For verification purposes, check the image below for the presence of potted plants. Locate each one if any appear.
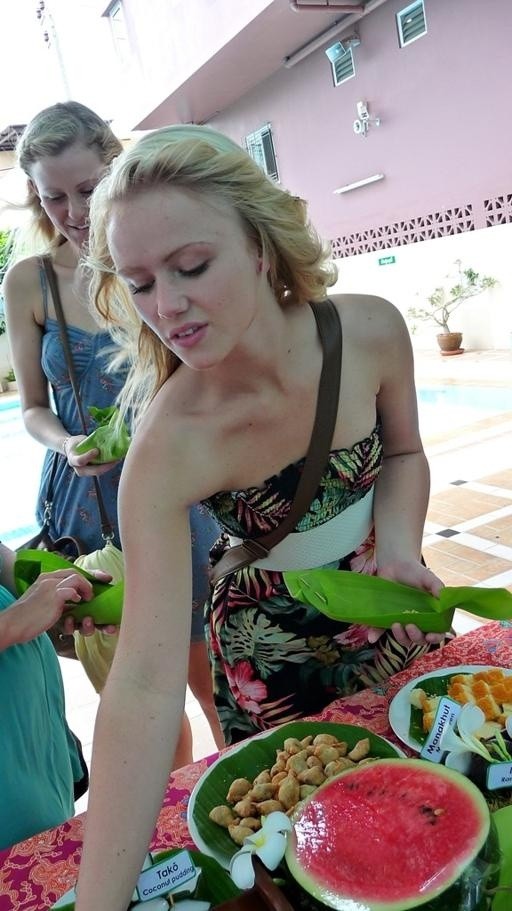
[404,260,495,350]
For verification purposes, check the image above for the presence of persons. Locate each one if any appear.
[3,100,238,774]
[73,124,446,911]
[0,537,118,857]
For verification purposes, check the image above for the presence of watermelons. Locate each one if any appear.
[285,757,501,911]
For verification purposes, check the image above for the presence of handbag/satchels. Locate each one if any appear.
[14,525,78,660]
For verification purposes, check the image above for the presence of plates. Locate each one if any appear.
[389,665,512,755]
[187,722,407,878]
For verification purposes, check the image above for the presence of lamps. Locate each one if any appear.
[325,33,360,63]
